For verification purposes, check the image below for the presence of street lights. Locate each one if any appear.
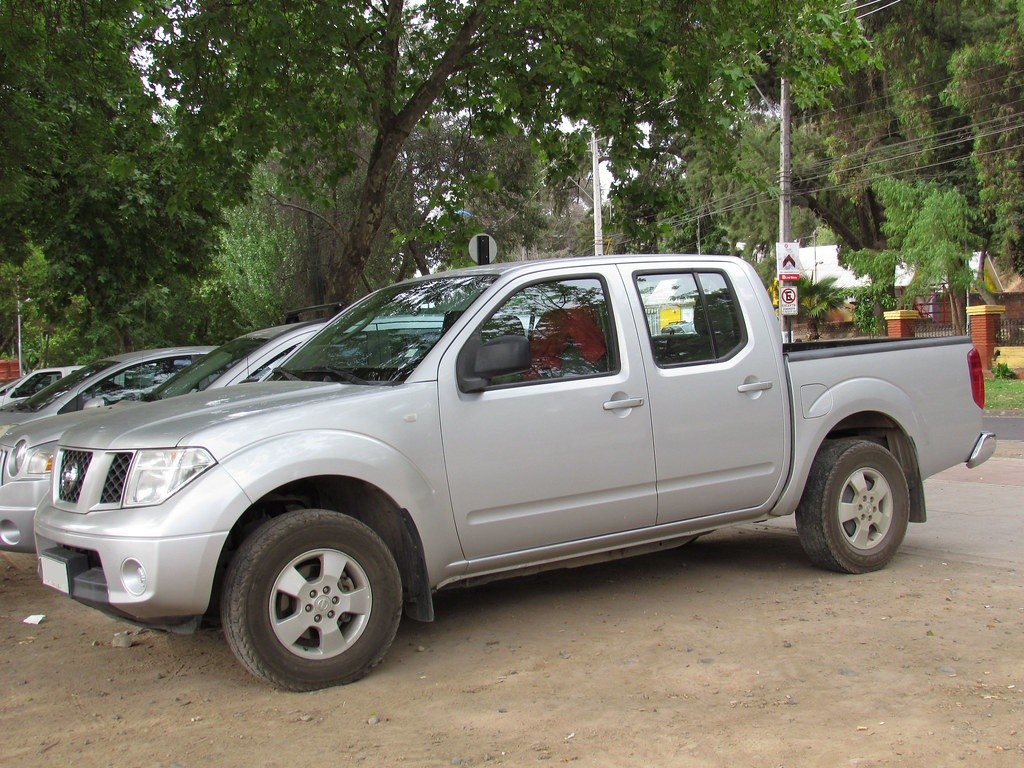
[17,285,34,376]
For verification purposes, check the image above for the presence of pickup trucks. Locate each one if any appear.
[33,253,999,693]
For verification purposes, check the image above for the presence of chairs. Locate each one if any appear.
[530,309,605,378]
[475,335,528,384]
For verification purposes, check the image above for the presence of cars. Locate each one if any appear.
[661,320,693,335]
[0,310,544,552]
[0,345,219,440]
[0,366,137,408]
